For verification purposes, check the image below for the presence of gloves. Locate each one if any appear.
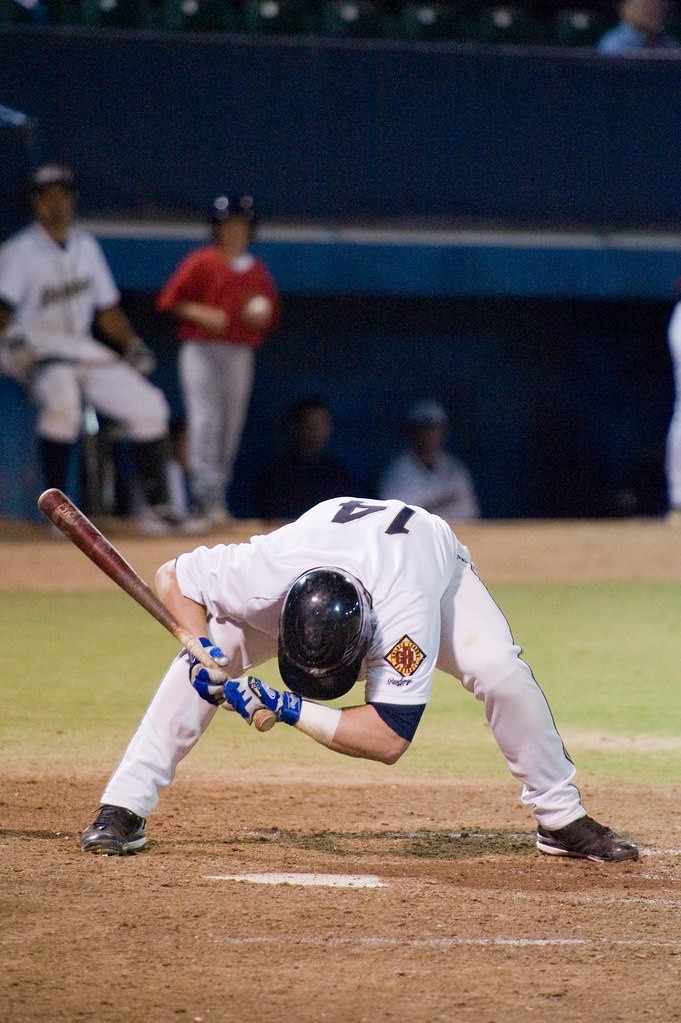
[187,637,229,706]
[6,332,35,364]
[225,676,301,727]
[124,341,155,374]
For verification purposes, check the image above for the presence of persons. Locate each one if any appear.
[378,397,481,519]
[158,198,277,533]
[0,163,213,534]
[270,395,378,518]
[76,495,640,863]
[598,0,679,52]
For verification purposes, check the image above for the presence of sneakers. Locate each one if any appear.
[79,804,149,854]
[537,817,637,863]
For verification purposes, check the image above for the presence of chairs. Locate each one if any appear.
[0,0,605,48]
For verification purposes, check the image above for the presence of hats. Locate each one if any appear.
[410,401,446,422]
[32,165,77,191]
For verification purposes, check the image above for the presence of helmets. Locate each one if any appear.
[277,565,372,700]
[210,186,260,237]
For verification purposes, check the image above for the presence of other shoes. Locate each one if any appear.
[136,508,212,536]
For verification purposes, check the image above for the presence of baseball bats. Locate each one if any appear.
[36,487,277,732]
